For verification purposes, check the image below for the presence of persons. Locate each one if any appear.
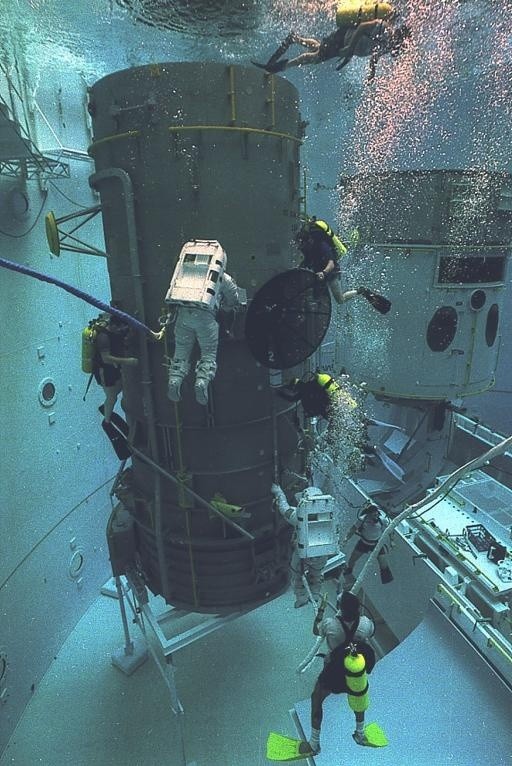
[271,481,328,608]
[93,315,138,423]
[167,272,240,405]
[306,464,314,486]
[342,506,396,575]
[280,19,412,86]
[293,220,364,304]
[308,591,376,756]
[275,373,379,454]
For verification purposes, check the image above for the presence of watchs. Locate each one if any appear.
[322,270,328,277]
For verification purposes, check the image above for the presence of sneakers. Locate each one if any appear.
[167,379,180,402]
[356,732,364,741]
[383,570,391,577]
[362,287,366,295]
[102,419,112,427]
[308,596,318,604]
[295,599,308,608]
[193,382,208,404]
[371,447,375,454]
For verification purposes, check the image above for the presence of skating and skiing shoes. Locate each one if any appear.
[298,742,317,753]
